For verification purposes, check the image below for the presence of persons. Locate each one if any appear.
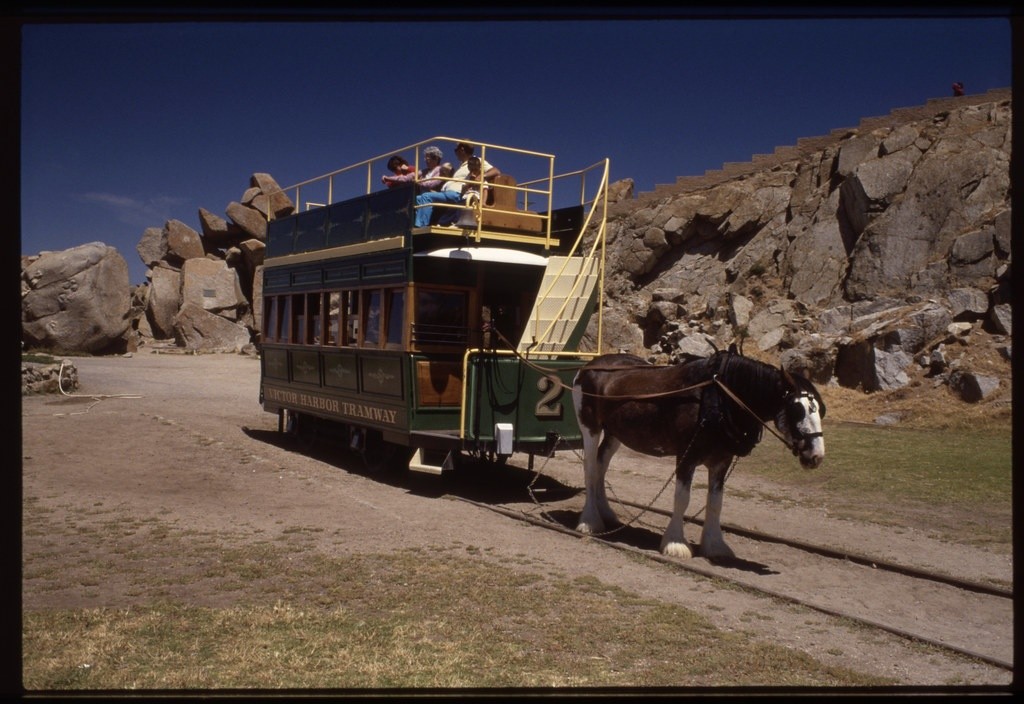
[415,136,500,227]
[381,146,443,195]
[449,157,489,230]
[440,161,454,183]
[385,155,416,188]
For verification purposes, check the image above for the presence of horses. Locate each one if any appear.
[572,350,827,560]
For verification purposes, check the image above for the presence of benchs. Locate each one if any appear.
[454,175,542,233]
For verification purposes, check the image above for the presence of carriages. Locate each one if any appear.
[259,135,827,563]
